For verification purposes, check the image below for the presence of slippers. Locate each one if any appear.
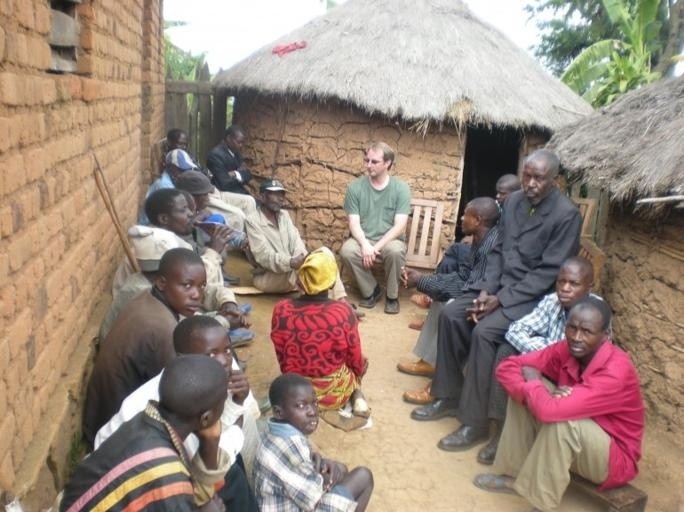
[474,474,518,494]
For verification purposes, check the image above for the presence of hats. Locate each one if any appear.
[165,148,196,170]
[259,178,287,193]
[175,172,215,196]
[296,250,337,293]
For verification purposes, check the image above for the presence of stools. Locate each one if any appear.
[567,469,648,511]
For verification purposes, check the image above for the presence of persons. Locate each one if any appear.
[408,148,582,451]
[478,256,611,464]
[474,296,645,509]
[248,374,373,510]
[269,246,372,422]
[59,121,256,511]
[399,173,521,404]
[337,139,411,316]
[241,176,362,323]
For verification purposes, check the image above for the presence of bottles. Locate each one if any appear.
[191,217,248,250]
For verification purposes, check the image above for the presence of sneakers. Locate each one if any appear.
[228,329,253,348]
[354,397,370,418]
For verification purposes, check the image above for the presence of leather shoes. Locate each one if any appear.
[360,286,382,306]
[478,434,499,463]
[438,425,489,452]
[386,296,399,314]
[399,358,457,420]
[410,319,424,329]
[411,294,432,308]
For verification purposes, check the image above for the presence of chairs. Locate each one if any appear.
[341,194,445,302]
[568,196,596,240]
[575,237,604,290]
